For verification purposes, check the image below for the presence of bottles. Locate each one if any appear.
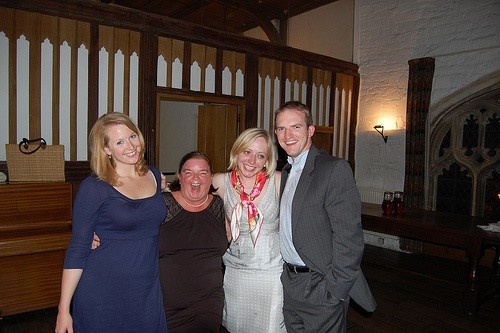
[381,191,407,218]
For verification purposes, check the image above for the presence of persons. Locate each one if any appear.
[55,112,168,333]
[91,151,232,333]
[274,101,377,333]
[161,127,287,333]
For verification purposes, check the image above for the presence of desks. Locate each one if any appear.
[360,202,500,321]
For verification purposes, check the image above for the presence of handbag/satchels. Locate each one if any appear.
[5,137,66,182]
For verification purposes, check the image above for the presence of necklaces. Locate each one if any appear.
[182,195,209,207]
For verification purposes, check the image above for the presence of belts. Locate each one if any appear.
[288,265,316,273]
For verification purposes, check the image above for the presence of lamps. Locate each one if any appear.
[374,125,388,144]
